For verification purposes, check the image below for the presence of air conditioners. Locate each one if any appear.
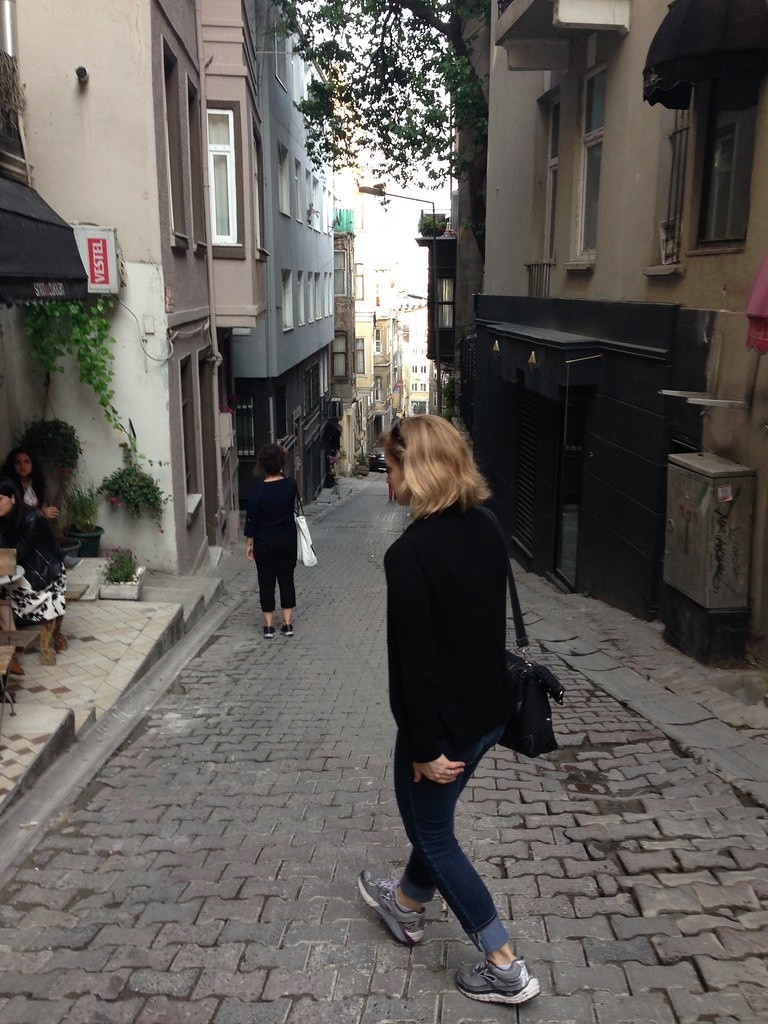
[69,221,121,296]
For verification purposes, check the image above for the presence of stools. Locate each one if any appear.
[0,644,18,716]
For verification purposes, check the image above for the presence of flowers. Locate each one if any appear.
[95,543,135,582]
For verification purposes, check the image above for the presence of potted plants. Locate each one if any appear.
[354,452,371,476]
[60,477,104,557]
[46,489,82,557]
[417,216,448,236]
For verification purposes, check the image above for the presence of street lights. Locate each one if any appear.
[357,186,443,418]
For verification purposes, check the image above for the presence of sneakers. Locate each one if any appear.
[455,956,540,1004]
[280,624,294,637]
[263,625,277,638]
[64,557,84,571]
[357,868,423,943]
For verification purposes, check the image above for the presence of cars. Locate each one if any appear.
[369,448,387,471]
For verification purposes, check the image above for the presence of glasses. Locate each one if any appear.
[392,420,407,450]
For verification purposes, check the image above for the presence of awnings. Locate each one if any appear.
[0,170,88,302]
[643,0,768,110]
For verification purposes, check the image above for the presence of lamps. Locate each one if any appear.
[491,339,509,361]
[526,350,546,375]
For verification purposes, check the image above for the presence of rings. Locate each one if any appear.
[450,770,455,776]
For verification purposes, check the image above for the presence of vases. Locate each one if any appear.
[100,564,148,599]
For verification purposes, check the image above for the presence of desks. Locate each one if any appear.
[0,582,90,666]
[0,564,26,586]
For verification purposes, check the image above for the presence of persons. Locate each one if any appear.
[243,444,297,638]
[0,449,67,675]
[356,414,541,1004]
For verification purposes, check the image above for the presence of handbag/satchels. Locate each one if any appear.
[497,647,566,758]
[296,514,317,567]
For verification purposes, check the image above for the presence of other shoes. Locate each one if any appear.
[389,500,391,502]
